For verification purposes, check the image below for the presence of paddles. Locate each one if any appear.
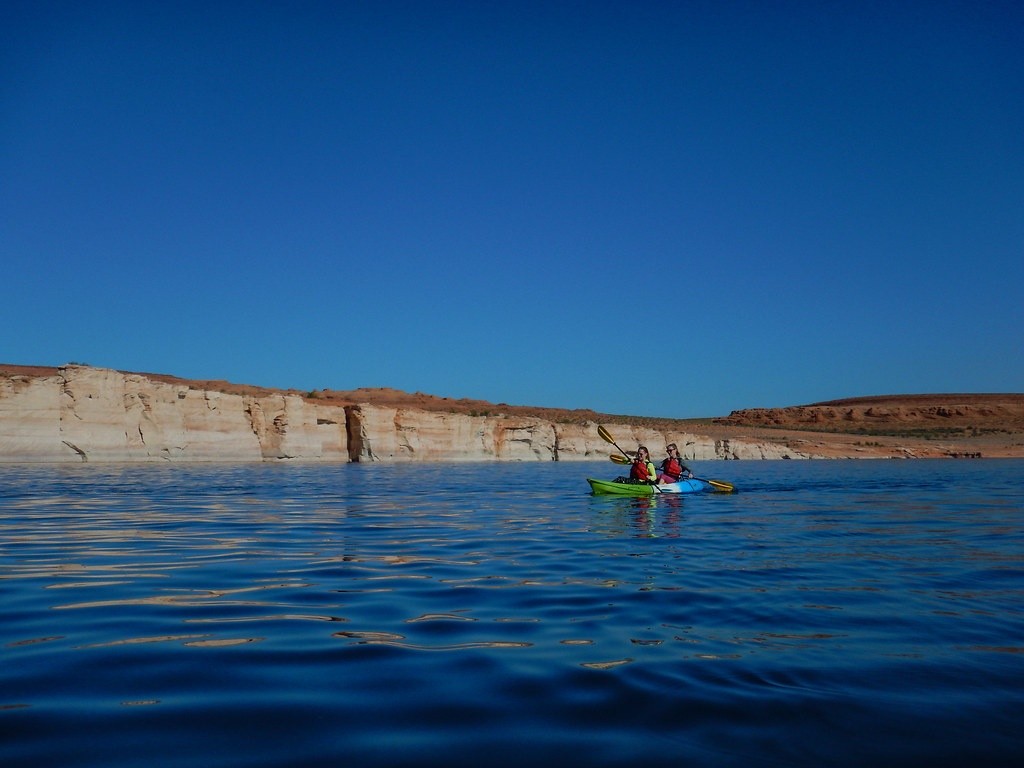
[597,425,668,497]
[610,454,733,493]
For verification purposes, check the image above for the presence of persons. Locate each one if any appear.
[659,443,693,485]
[611,447,657,485]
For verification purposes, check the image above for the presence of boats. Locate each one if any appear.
[586,477,705,499]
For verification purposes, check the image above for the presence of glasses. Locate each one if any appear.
[637,453,643,456]
[667,449,675,452]
